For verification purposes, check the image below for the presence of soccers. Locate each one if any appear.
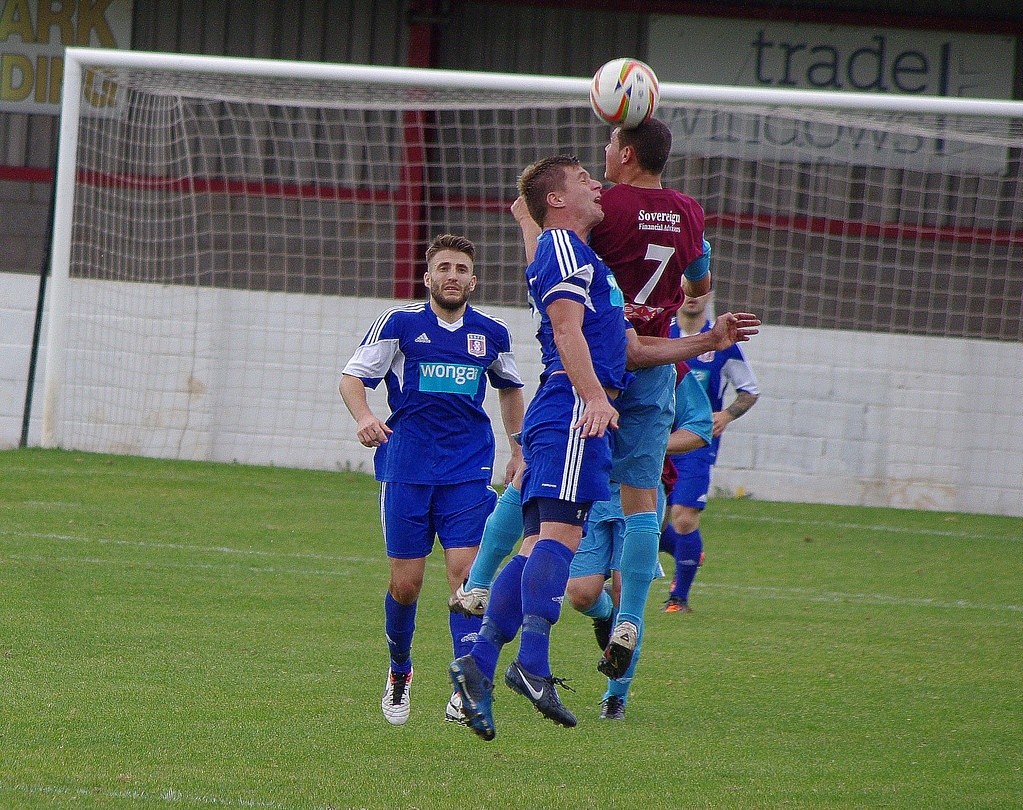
[588,56,661,130]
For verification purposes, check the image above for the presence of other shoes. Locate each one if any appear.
[661,595,688,613]
[669,551,705,597]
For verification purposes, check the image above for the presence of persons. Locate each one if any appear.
[565,359,714,721]
[450,154,761,742]
[657,289,761,611]
[449,118,711,680]
[340,234,525,726]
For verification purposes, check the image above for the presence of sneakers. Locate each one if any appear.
[591,583,614,652]
[449,654,496,741]
[598,696,625,722]
[447,578,488,620]
[597,620,637,681]
[381,666,413,726]
[445,691,471,728]
[504,657,577,728]
